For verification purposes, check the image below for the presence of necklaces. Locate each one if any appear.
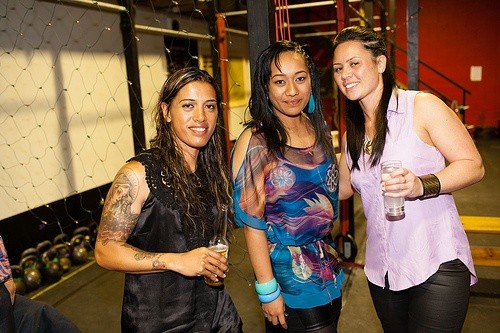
[363,95,383,153]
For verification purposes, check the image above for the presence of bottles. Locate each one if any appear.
[203,203,229,286]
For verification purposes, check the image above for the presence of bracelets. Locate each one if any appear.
[254,278,281,305]
[416,172,440,201]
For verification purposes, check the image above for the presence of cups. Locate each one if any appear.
[381,160,405,221]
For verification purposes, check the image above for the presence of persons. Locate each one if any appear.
[12,293,83,332]
[0,235,18,333]
[230,41,351,332]
[95,67,245,332]
[332,26,486,333]
